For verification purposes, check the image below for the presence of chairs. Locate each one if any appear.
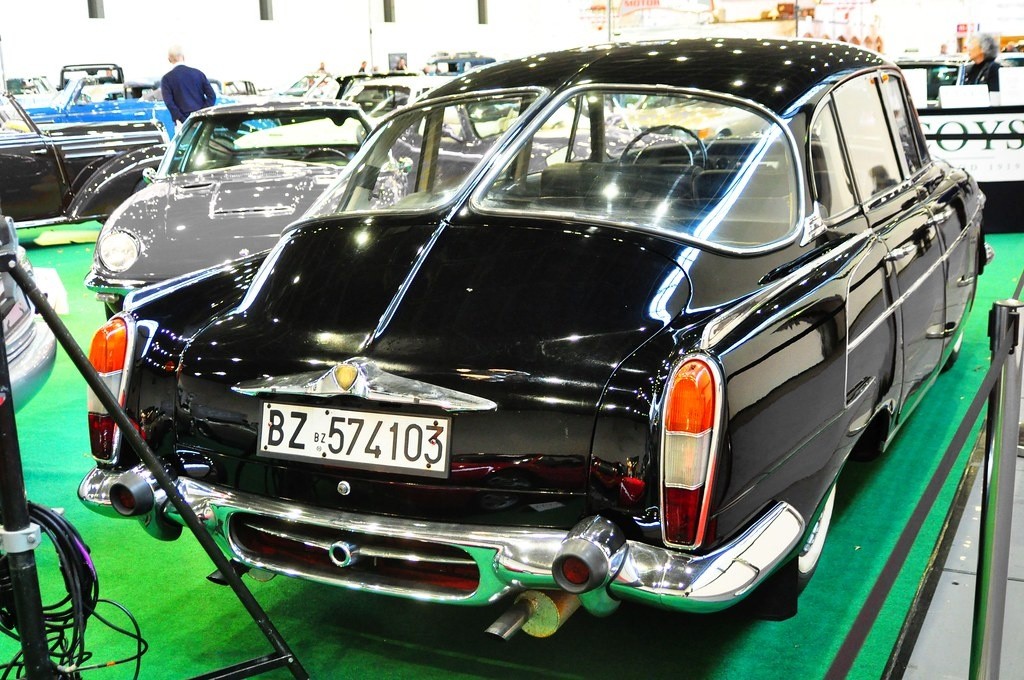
[545,163,703,205]
[690,169,850,203]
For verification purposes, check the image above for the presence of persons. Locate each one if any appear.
[964,33,1005,91]
[161,45,217,144]
[359,58,407,72]
[319,62,325,70]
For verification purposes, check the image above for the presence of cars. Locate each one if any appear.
[612,96,772,139]
[22,76,276,142]
[891,52,1024,107]
[0,91,185,230]
[231,75,645,166]
[82,99,412,322]
[75,37,994,641]
